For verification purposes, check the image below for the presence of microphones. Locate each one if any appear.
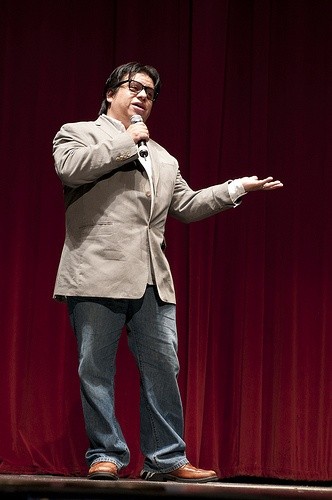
[130,115,148,158]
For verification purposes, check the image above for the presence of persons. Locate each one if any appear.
[52,62,283,483]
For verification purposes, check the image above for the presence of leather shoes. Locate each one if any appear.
[140,462,218,483]
[87,462,119,480]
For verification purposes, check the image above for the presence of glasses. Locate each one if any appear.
[114,79,159,101]
